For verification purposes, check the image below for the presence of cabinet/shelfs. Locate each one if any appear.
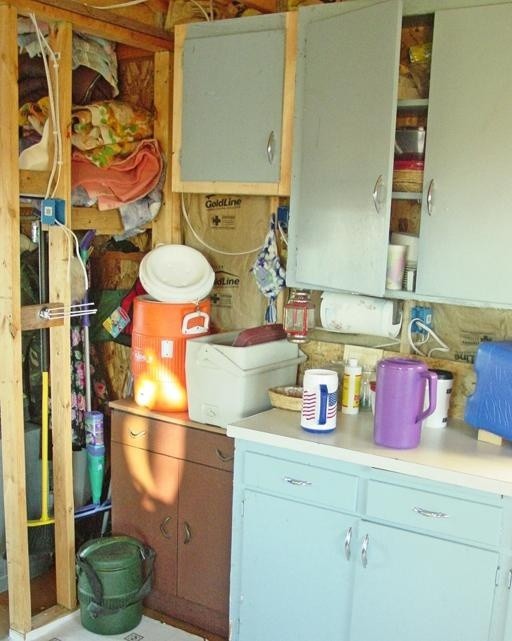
[170,11,292,199]
[286,0,512,312]
[108,398,235,641]
[229,438,511,639]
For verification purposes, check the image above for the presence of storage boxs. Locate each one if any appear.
[184,329,308,429]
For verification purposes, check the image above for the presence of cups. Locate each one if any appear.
[373,357,438,450]
[302,368,338,434]
[421,370,454,430]
[361,379,373,411]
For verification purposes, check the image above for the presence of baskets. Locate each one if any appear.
[392,169,424,193]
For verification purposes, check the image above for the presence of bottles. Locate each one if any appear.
[342,358,362,415]
[386,245,415,291]
[283,292,315,343]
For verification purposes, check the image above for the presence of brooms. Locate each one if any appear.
[4,218,57,560]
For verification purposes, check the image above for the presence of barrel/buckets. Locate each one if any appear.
[131,243,216,414]
[76,536,146,634]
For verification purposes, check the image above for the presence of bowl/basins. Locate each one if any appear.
[390,231,420,262]
